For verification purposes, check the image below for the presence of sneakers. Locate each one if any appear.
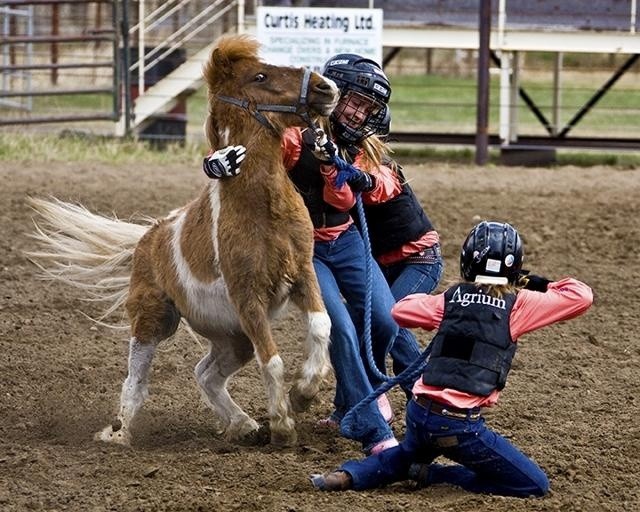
[372,438,398,456]
[376,393,392,423]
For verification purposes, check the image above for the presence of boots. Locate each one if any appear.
[312,470,351,490]
[407,462,438,483]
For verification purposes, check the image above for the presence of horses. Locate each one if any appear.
[19,34,342,450]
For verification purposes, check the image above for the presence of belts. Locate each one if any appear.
[412,395,481,422]
[403,244,441,265]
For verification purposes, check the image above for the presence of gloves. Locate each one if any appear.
[202,145,246,178]
[303,129,338,165]
[329,153,375,194]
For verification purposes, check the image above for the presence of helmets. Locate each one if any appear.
[460,222,522,287]
[366,105,390,138]
[322,53,391,143]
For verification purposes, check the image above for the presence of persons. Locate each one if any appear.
[201,53,401,454]
[309,221,595,496]
[315,103,441,429]
